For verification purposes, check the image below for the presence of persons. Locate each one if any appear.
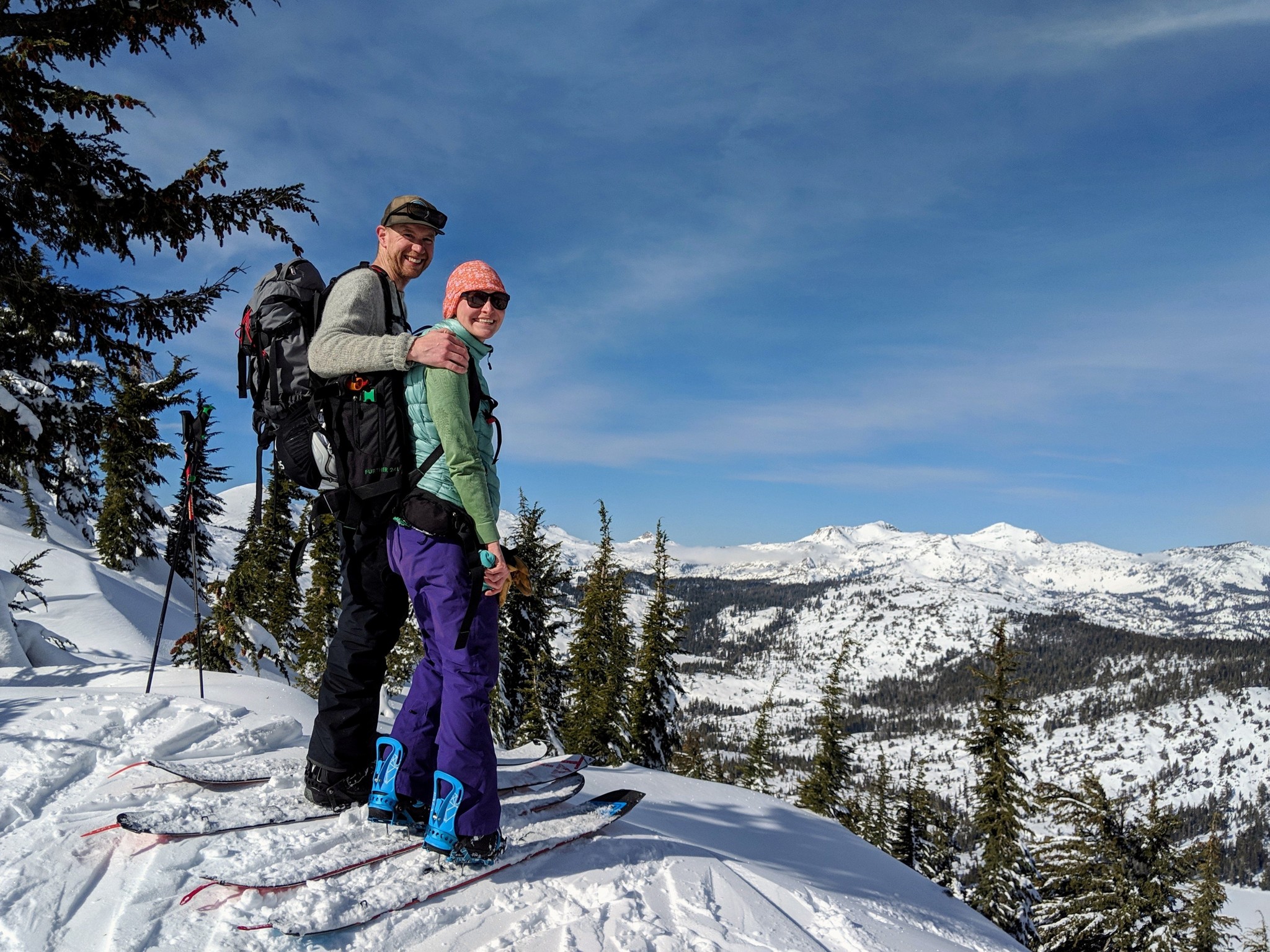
[367,258,515,868]
[304,195,471,813]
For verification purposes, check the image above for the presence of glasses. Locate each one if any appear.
[383,199,448,229]
[460,290,511,311]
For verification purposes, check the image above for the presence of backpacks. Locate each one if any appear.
[324,332,502,527]
[237,257,405,490]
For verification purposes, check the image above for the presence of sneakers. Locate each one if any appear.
[368,791,433,825]
[303,760,376,812]
[425,833,507,865]
[376,731,391,758]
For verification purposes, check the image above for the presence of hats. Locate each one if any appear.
[381,195,446,236]
[442,260,506,320]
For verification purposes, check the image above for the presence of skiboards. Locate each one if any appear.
[113,738,597,836]
[185,774,649,938]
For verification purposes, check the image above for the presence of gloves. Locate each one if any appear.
[499,555,533,607]
